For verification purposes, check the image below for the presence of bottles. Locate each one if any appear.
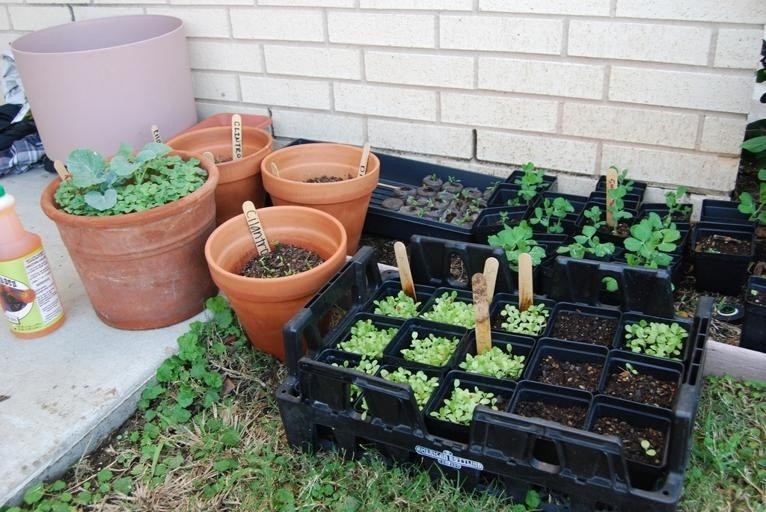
[0,179,68,339]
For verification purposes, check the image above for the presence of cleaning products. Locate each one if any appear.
[0,183,63,338]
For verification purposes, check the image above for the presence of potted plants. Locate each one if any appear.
[42,141,220,330]
